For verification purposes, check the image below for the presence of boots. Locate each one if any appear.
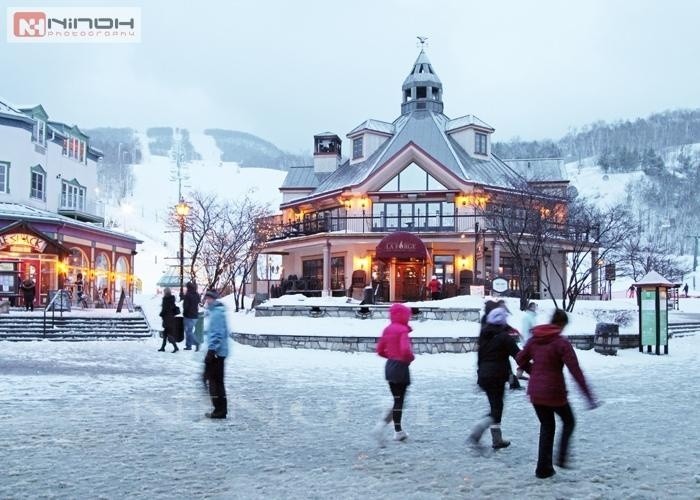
[466,424,484,446]
[158,340,167,352]
[491,426,510,448]
[171,343,178,353]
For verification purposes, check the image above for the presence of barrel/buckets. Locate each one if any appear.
[594,323,620,354]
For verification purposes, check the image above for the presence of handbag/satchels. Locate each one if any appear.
[173,305,180,315]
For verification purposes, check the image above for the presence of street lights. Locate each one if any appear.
[174,195,190,302]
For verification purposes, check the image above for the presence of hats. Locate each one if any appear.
[486,307,507,326]
[205,289,218,298]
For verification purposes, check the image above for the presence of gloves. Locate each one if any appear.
[205,349,215,364]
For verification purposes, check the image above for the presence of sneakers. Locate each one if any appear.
[206,412,225,418]
[372,425,387,447]
[394,430,408,440]
[184,346,191,350]
[511,386,525,390]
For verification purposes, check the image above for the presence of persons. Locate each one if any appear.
[460,307,532,454]
[22,275,36,311]
[159,288,180,353]
[513,310,603,479]
[369,303,414,448]
[200,289,230,419]
[428,274,442,300]
[481,298,540,394]
[683,283,689,298]
[182,281,202,353]
[627,285,636,298]
